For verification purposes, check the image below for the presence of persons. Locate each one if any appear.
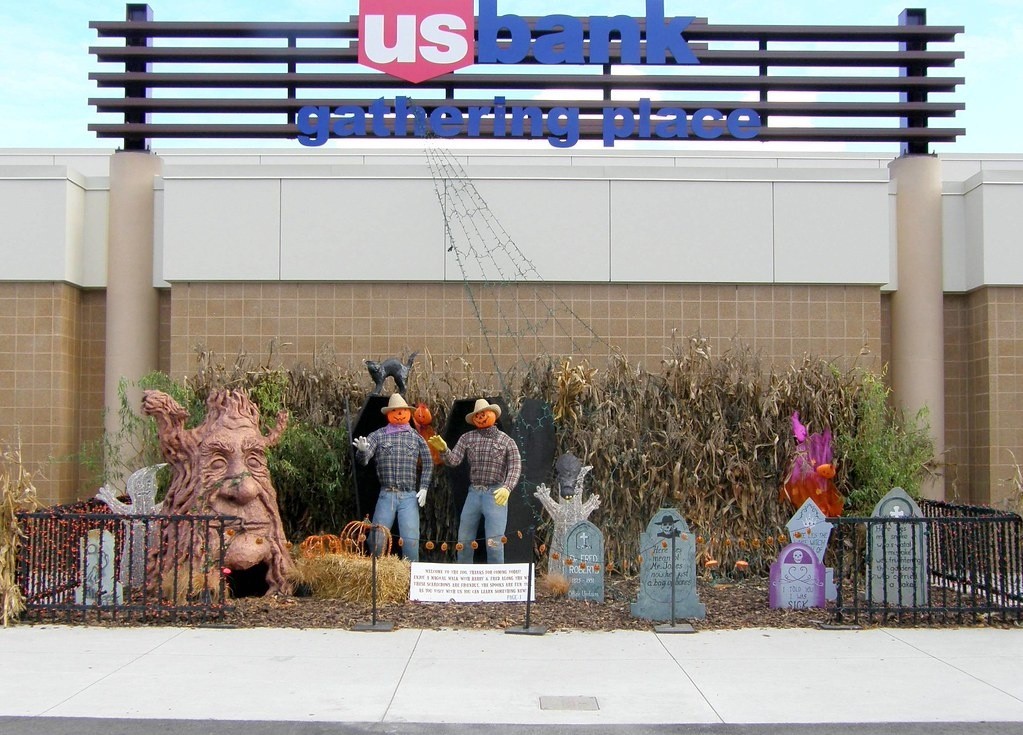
[352,392,433,562]
[426,398,521,564]
[141,383,299,597]
